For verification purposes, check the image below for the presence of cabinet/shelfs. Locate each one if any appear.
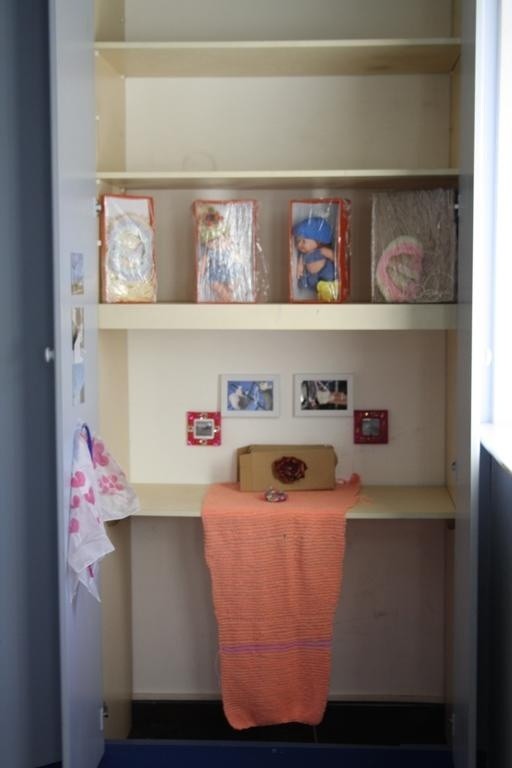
[43,0,482,768]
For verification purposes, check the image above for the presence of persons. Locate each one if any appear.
[289,213,341,305]
[197,204,250,301]
